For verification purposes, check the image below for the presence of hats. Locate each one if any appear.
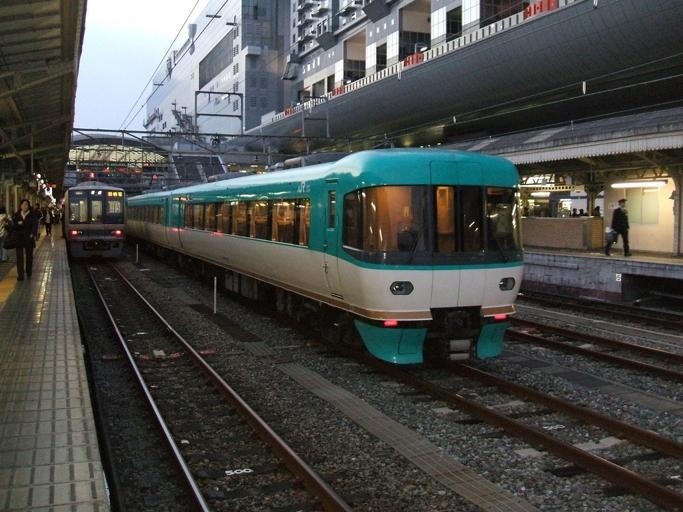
[618,199,629,202]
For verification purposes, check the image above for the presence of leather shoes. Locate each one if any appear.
[605,249,611,256]
[625,253,633,257]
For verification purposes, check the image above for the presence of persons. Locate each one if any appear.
[604,199,632,256]
[571,206,601,216]
[0,199,38,281]
[34,203,65,240]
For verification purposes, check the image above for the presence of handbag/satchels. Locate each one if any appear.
[605,231,618,242]
[2,230,36,249]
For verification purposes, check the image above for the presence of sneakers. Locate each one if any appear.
[16,271,32,282]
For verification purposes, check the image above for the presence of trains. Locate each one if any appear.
[127,147,525,366]
[62,181,127,265]
